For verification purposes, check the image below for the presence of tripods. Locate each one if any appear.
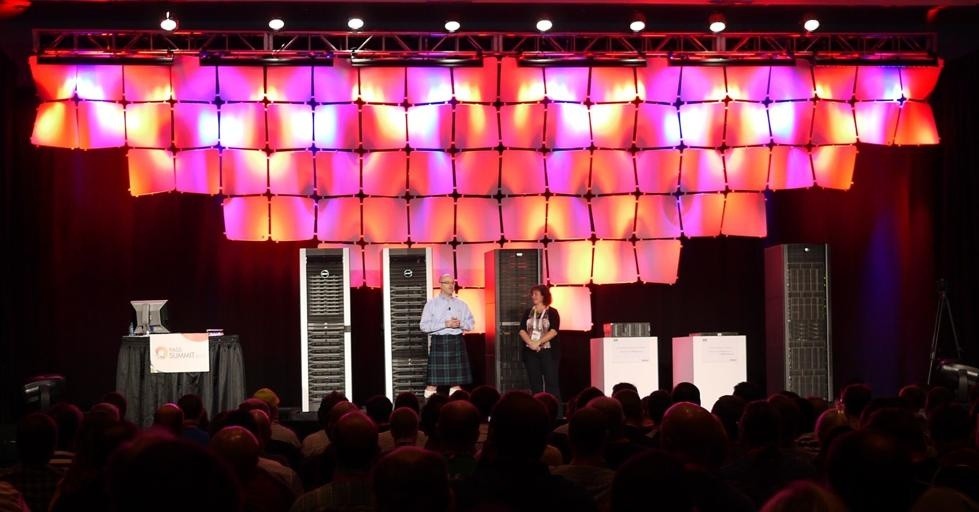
[921,297,963,403]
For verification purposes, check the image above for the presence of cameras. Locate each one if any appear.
[935,273,950,291]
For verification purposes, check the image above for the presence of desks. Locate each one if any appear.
[114,334,245,424]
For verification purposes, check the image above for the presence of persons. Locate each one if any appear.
[1,371,979,510]
[520,285,565,423]
[420,274,475,400]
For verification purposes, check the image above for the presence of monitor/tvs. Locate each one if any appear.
[129,298,172,335]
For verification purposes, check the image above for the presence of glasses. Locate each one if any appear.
[439,281,454,284]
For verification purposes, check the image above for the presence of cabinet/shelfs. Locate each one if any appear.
[672,335,748,415]
[590,338,658,399]
